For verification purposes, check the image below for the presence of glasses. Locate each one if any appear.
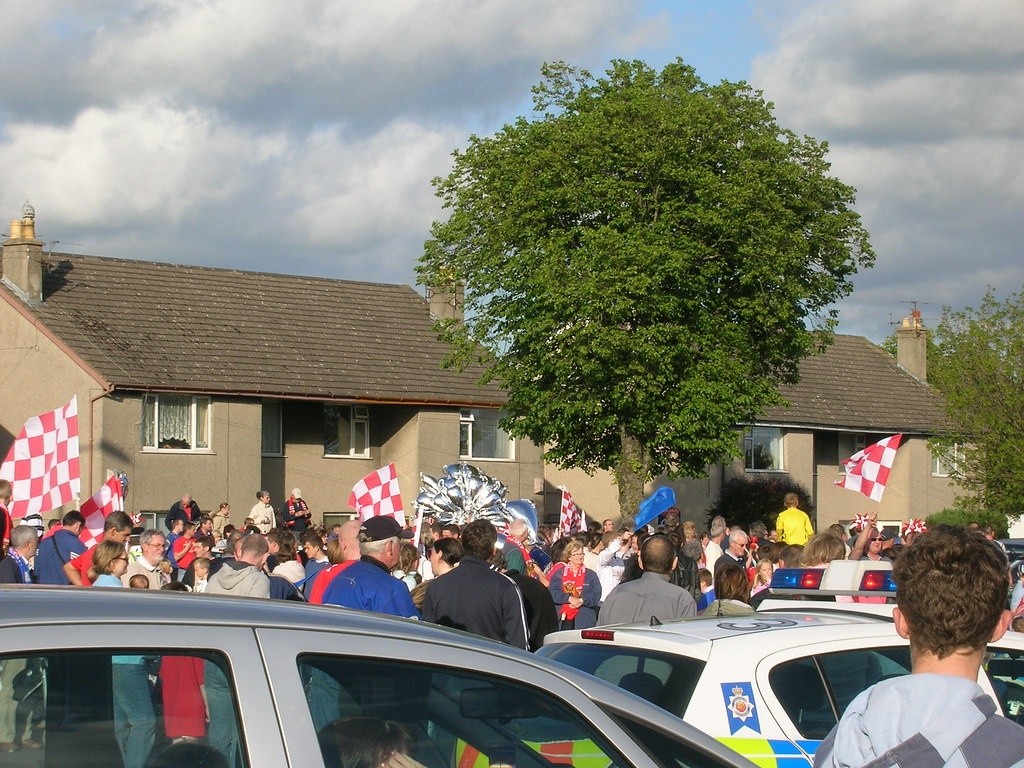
[573,553,586,557]
[869,537,883,542]
[734,542,749,547]
[115,557,129,563]
[144,542,166,549]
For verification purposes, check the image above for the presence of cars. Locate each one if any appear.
[996,537,1024,581]
[1,582,759,768]
[452,562,1024,768]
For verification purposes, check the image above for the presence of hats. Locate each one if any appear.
[591,532,603,549]
[357,515,415,542]
[292,488,302,499]
[130,527,146,538]
[683,540,705,561]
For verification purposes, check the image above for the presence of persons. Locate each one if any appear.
[812,523,1024,768]
[0,478,1024,768]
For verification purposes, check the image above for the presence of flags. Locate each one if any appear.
[0,394,81,520]
[560,484,582,539]
[79,473,124,550]
[633,485,677,532]
[832,433,902,502]
[346,462,407,527]
